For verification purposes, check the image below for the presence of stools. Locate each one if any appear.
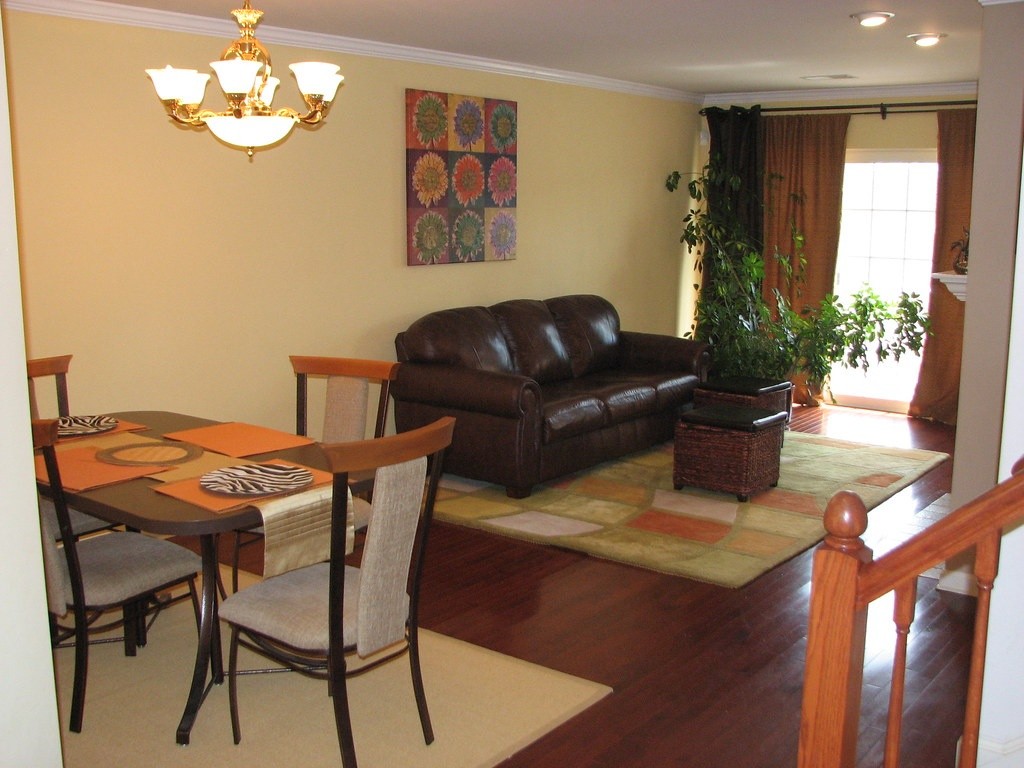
[673,404,788,503]
[691,375,793,448]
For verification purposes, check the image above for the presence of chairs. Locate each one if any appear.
[31,417,211,734]
[231,356,402,593]
[27,354,135,658]
[216,412,458,768]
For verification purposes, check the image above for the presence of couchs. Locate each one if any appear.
[389,295,713,499]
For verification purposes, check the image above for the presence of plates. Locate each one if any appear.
[54,415,120,437]
[199,462,313,496]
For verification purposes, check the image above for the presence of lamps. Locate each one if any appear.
[144,0,346,157]
[856,13,888,30]
[913,35,941,50]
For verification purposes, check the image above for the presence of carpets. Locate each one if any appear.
[348,428,950,589]
[52,526,614,768]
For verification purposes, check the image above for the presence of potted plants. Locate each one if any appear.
[665,150,936,423]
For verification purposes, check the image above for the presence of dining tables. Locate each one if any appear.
[32,412,376,748]
